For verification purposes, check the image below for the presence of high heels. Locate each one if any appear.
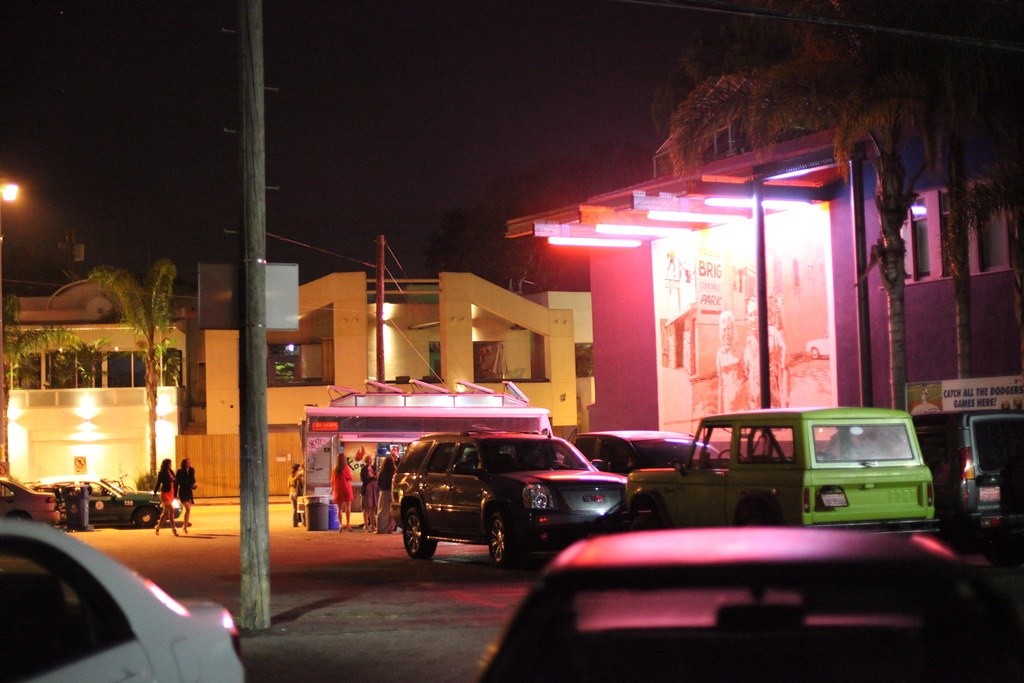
[363,525,373,533]
[346,524,353,532]
[370,524,376,532]
[338,524,343,533]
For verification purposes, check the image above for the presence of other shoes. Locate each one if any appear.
[172,529,179,536]
[155,525,159,535]
[183,525,188,533]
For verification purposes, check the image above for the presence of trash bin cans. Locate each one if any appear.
[304,495,329,530]
[62,484,91,532]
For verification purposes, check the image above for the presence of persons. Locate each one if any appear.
[744,297,789,410]
[330,452,355,533]
[154,458,180,536]
[360,455,378,533]
[287,463,305,527]
[716,310,749,415]
[377,456,399,534]
[174,458,196,533]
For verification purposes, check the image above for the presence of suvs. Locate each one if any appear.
[639,408,939,534]
[828,407,1023,539]
[392,427,632,565]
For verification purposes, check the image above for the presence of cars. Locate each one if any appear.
[492,525,1020,682]
[0,477,61,528]
[48,479,182,529]
[572,431,727,483]
[0,518,248,683]
